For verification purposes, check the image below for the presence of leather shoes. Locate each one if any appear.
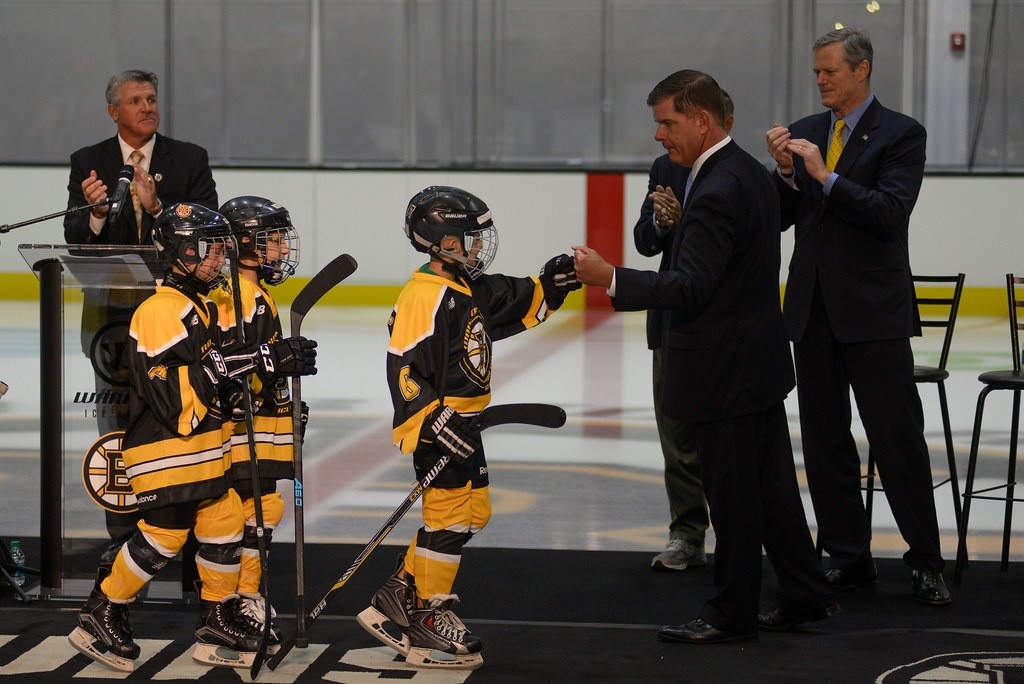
[911,567,952,605]
[825,558,878,590]
[758,592,837,631]
[656,615,733,642]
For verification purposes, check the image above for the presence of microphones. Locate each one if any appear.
[109,164,134,225]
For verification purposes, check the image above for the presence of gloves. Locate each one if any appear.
[263,337,319,382]
[208,341,262,386]
[429,408,481,466]
[538,253,582,296]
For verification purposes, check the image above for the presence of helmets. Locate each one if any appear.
[218,196,302,287]
[151,201,240,297]
[403,184,501,280]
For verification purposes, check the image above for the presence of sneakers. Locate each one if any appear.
[68,568,142,673]
[651,539,708,571]
[356,557,416,657]
[191,579,283,669]
[405,586,484,670]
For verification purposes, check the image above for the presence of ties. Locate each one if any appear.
[129,151,148,243]
[683,169,695,212]
[825,120,847,171]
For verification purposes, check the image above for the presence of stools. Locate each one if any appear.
[814,272,966,558]
[950,273,1024,593]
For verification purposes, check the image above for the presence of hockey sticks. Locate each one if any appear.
[290,253,358,651]
[0,567,34,606]
[266,402,566,671]
[1,560,41,574]
[229,245,272,680]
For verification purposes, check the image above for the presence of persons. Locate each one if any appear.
[358,185,582,670]
[68,200,260,671]
[64,69,219,608]
[193,197,318,668]
[766,29,951,606]
[573,70,822,641]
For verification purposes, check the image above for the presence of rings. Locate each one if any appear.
[668,206,671,211]
[799,146,802,149]
[148,177,151,182]
